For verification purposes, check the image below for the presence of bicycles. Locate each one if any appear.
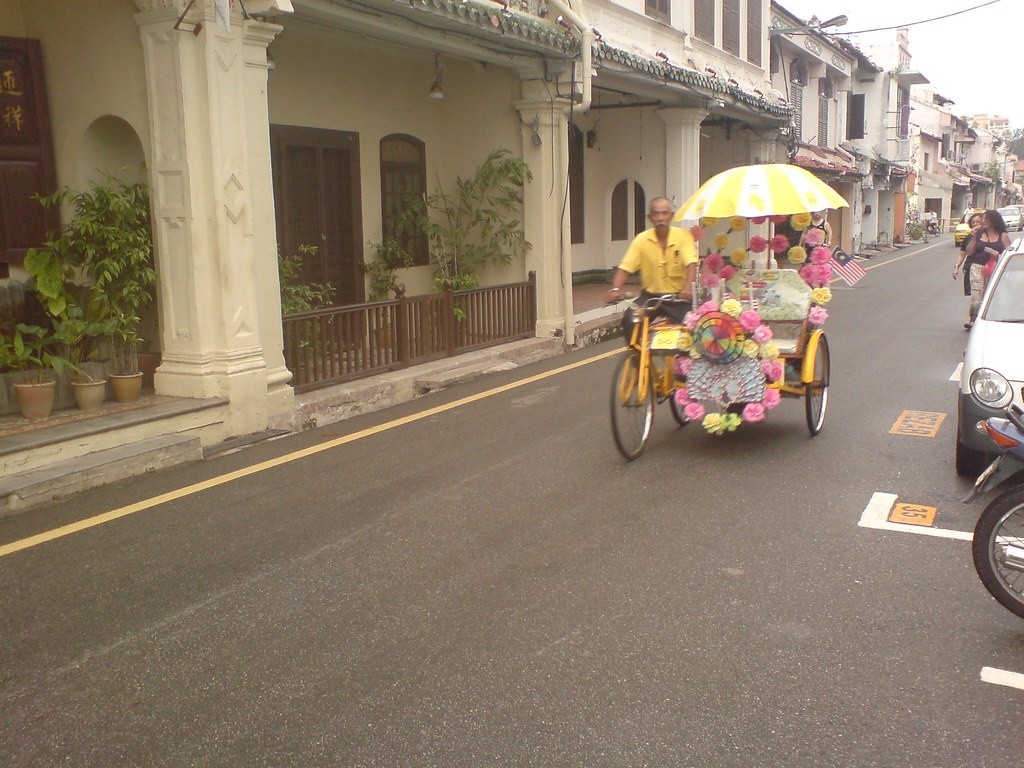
[603,290,696,461]
[922,219,941,237]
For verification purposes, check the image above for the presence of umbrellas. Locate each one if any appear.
[672,163,849,269]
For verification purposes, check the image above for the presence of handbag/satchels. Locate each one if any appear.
[981,254,997,277]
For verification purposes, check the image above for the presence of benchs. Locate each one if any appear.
[727,270,813,356]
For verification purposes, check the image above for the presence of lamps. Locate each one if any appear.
[428,52,448,99]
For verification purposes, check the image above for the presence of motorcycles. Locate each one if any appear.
[962,403,1024,620]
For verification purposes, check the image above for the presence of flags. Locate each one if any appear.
[828,247,866,286]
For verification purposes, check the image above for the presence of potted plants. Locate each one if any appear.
[908,228,924,240]
[32,161,157,403]
[357,233,416,351]
[1,321,56,420]
[274,241,336,386]
[25,244,118,415]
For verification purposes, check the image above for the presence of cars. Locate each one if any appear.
[953,208,986,247]
[997,204,1024,232]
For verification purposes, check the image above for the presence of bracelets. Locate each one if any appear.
[609,287,619,292]
[955,267,959,269]
[955,265,960,267]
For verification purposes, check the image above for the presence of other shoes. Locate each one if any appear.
[964,324,972,329]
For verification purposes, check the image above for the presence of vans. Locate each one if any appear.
[955,237,1024,477]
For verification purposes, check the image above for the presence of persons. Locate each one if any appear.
[953,213,984,322]
[964,209,1010,328]
[930,211,937,229]
[963,204,974,223]
[799,212,832,264]
[605,197,697,389]
